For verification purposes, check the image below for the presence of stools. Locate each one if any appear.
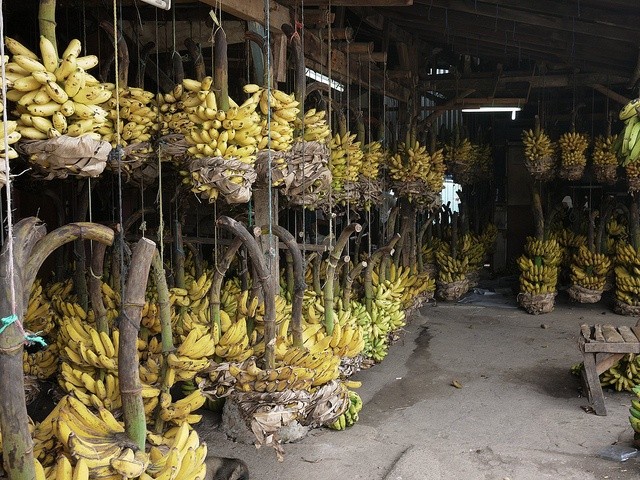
[577,325,640,416]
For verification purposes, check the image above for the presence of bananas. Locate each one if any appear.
[22,273,212,480]
[521,128,557,182]
[362,264,421,366]
[390,141,447,211]
[594,133,618,184]
[568,232,614,304]
[186,83,300,202]
[445,138,494,186]
[103,84,188,173]
[558,132,591,182]
[570,354,640,392]
[213,279,294,395]
[516,228,567,314]
[1,36,110,182]
[628,386,640,433]
[331,132,389,211]
[293,108,332,209]
[607,216,640,316]
[611,98,640,197]
[294,259,362,431]
[420,225,498,301]
